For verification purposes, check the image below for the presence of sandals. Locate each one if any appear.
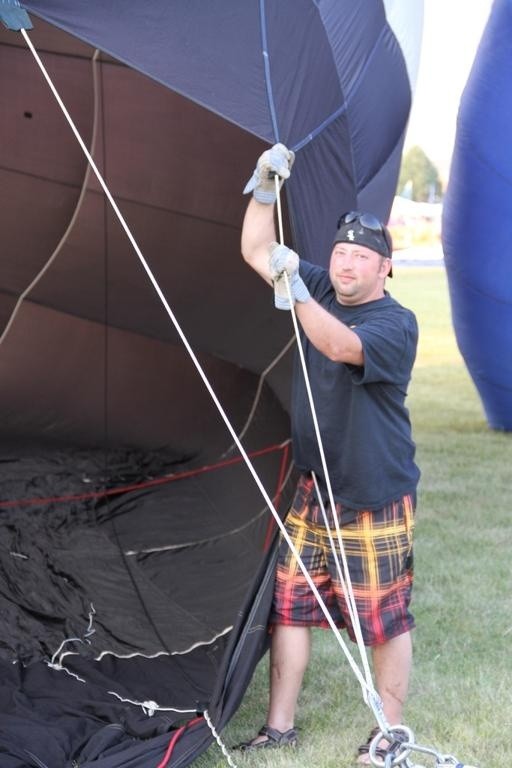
[358,725,411,768]
[228,724,301,753]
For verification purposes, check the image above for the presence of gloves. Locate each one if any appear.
[266,243,310,312]
[242,142,296,207]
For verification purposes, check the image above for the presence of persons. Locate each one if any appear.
[231,141,423,767]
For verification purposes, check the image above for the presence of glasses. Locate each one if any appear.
[335,209,393,257]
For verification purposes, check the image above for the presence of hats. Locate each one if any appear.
[330,210,397,280]
